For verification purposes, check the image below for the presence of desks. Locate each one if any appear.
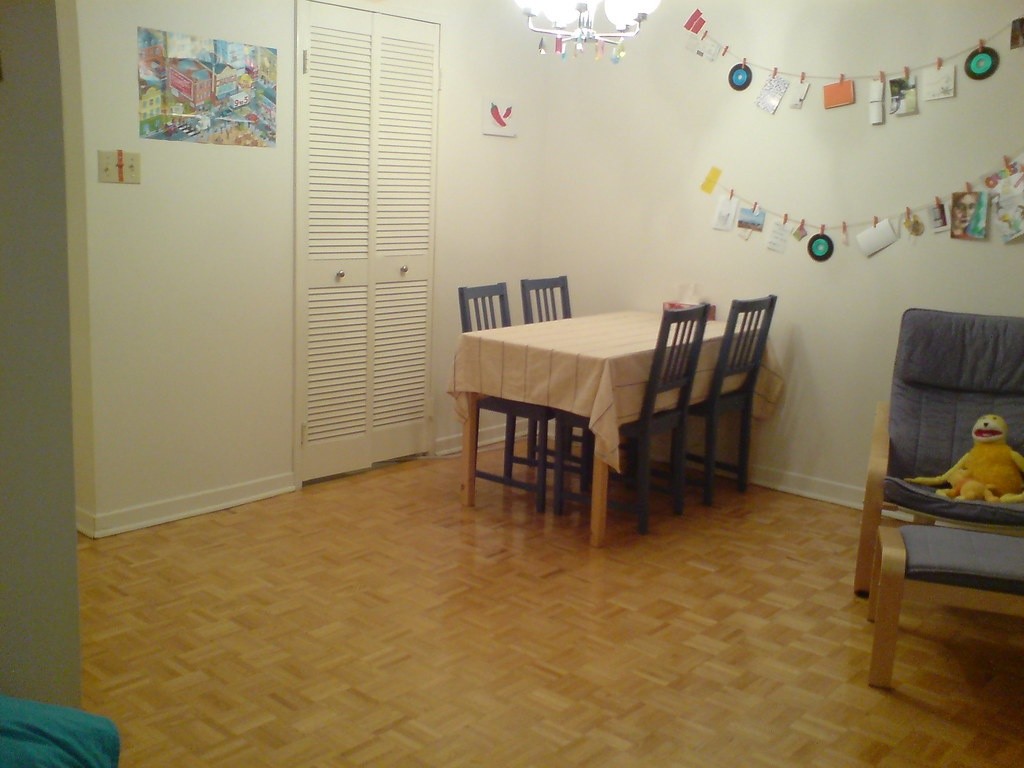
[447,307,758,544]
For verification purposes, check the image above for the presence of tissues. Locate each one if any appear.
[662,299,715,321]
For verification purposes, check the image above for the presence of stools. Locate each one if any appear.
[866,525,1024,689]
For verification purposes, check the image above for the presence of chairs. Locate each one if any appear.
[521,275,632,481]
[657,292,777,506]
[553,300,712,535]
[457,282,556,513]
[852,307,1024,596]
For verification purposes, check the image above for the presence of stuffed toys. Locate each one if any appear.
[903,415,1024,504]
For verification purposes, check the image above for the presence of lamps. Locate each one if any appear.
[515,0,660,60]
